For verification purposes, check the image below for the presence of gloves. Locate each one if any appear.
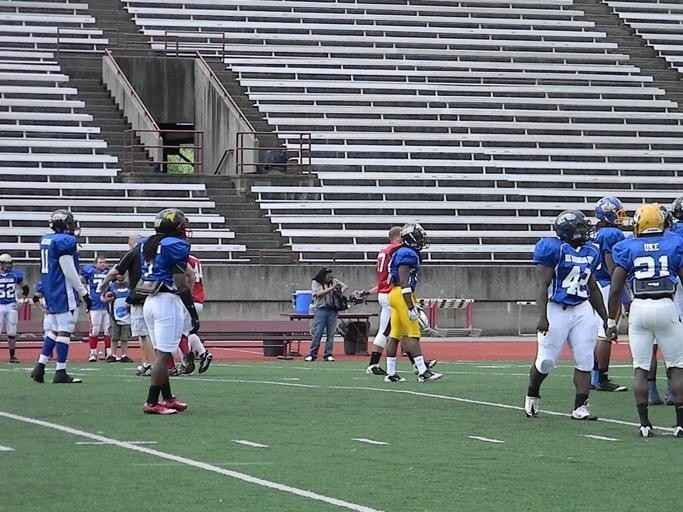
[359,290,370,296]
[17,299,25,309]
[84,294,92,313]
[408,308,420,321]
[189,310,201,335]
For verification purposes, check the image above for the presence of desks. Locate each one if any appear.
[281,312,378,356]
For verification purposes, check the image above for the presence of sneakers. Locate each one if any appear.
[120,356,135,364]
[414,359,439,375]
[571,400,599,421]
[673,425,683,437]
[639,426,652,438]
[168,367,179,378]
[107,355,116,363]
[89,348,98,362]
[33,365,47,384]
[595,382,629,392]
[53,369,83,384]
[11,357,21,363]
[590,383,596,391]
[649,390,664,405]
[417,370,444,385]
[145,403,177,415]
[666,391,676,405]
[179,352,196,377]
[137,362,153,377]
[161,395,189,411]
[199,351,213,374]
[525,394,541,418]
[305,355,314,361]
[325,356,335,363]
[364,363,387,377]
[107,354,122,362]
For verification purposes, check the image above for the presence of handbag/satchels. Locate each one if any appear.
[331,285,350,311]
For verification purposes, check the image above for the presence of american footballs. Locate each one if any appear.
[105,291,114,300]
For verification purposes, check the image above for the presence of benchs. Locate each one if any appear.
[1,318,328,362]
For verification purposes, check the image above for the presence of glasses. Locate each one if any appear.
[326,269,333,272]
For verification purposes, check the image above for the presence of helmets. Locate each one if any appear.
[400,223,431,251]
[50,209,76,234]
[634,206,664,237]
[670,197,683,220]
[155,209,194,241]
[554,211,592,242]
[1,254,13,272]
[351,290,367,306]
[595,197,627,226]
[419,310,429,331]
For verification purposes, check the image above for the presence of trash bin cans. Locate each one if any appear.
[343,320,371,354]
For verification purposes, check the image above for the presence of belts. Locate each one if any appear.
[634,292,672,304]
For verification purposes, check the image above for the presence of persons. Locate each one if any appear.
[383,223,444,384]
[100,275,135,363]
[167,262,195,376]
[135,208,201,415]
[590,197,635,392]
[100,235,155,376]
[365,226,437,377]
[605,204,683,438]
[0,254,30,363]
[647,207,682,407]
[304,268,348,362]
[358,285,408,357]
[31,209,91,384]
[670,196,682,324]
[33,269,86,363]
[525,209,608,421]
[188,256,213,374]
[83,256,117,362]
